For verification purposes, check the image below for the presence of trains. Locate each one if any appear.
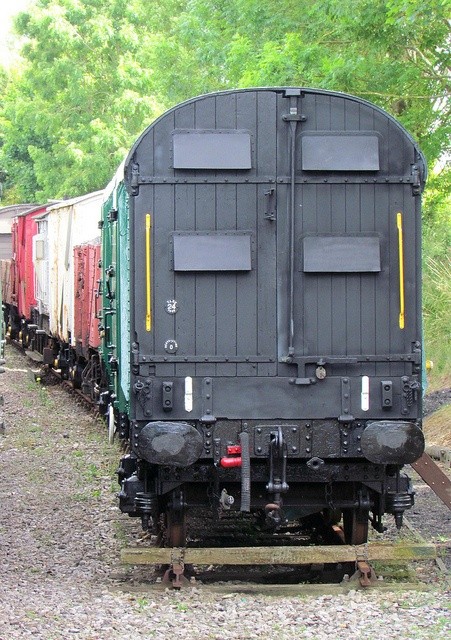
[0,86,439,585]
